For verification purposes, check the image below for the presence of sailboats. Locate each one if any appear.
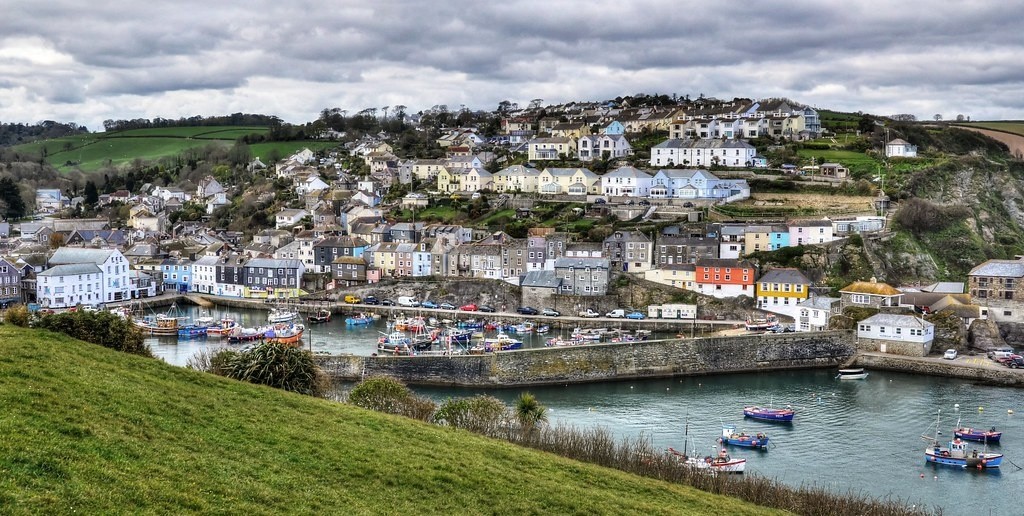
[267,261,299,323]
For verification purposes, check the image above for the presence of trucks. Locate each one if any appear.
[987,347,1014,362]
[83,306,97,312]
[579,309,599,318]
[517,307,537,315]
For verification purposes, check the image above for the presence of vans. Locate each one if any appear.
[606,309,624,318]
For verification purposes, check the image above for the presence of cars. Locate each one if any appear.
[542,308,560,317]
[943,349,958,360]
[478,305,495,313]
[626,311,645,319]
[345,295,478,311]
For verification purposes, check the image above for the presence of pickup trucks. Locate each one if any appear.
[998,355,1024,369]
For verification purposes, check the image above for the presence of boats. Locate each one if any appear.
[921,409,1003,469]
[667,405,795,474]
[954,413,1002,443]
[345,305,652,355]
[307,310,331,325]
[110,291,305,344]
[745,313,779,331]
[838,368,868,380]
